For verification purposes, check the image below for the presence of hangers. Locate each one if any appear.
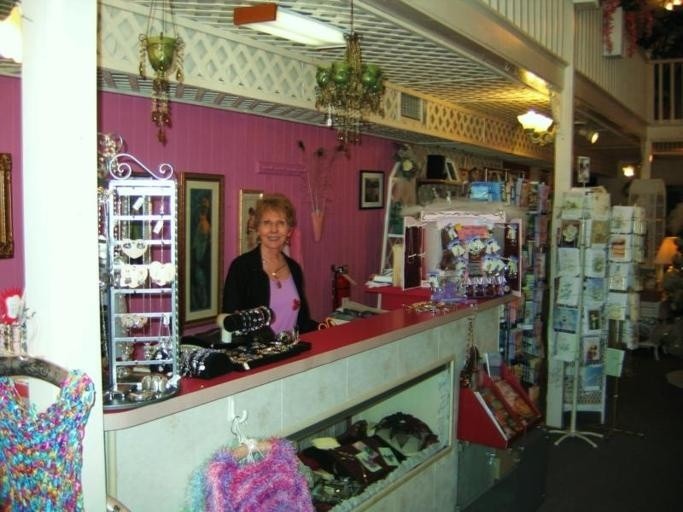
[0,296,94,403]
[206,415,299,487]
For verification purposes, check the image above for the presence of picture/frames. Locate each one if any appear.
[361,169,384,210]
[178,170,225,330]
[0,152,13,259]
[237,188,264,255]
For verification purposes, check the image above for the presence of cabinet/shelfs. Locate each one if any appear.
[96,152,185,411]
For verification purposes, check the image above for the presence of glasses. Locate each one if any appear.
[257,219,287,227]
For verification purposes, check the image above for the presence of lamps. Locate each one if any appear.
[653,237,683,293]
[139,0,186,143]
[621,161,641,178]
[313,0,392,155]
[515,109,557,147]
[232,3,350,52]
[576,129,600,144]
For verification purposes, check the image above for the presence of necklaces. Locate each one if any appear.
[178,338,225,380]
[261,258,288,277]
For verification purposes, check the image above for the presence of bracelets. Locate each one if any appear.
[234,305,271,335]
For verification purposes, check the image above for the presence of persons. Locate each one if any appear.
[222,192,321,344]
